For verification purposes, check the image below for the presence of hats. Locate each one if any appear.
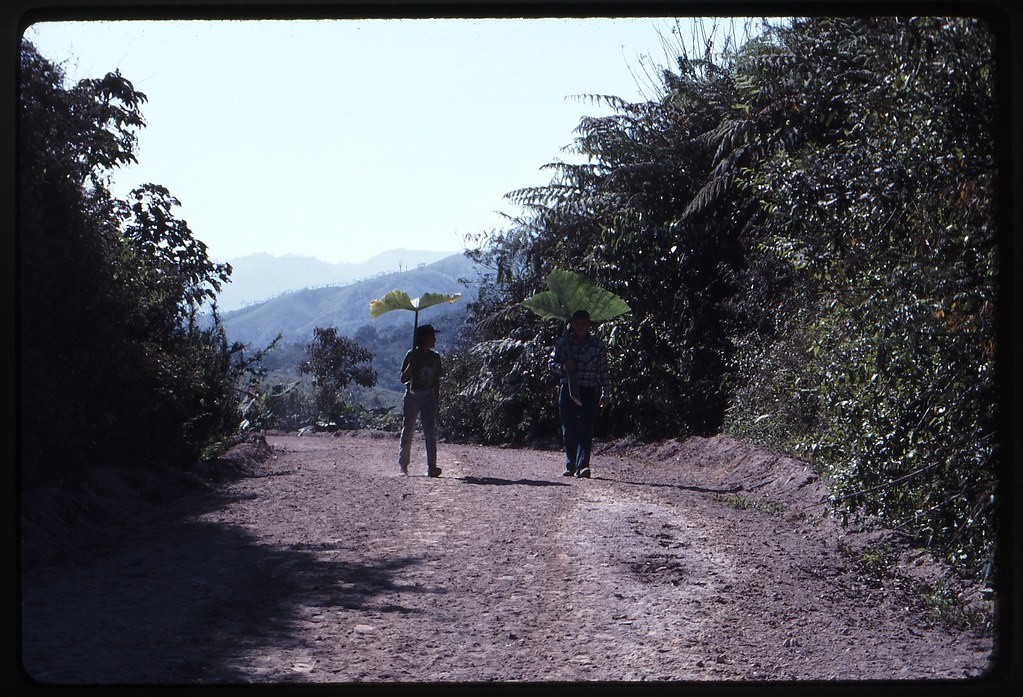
[417,325,440,337]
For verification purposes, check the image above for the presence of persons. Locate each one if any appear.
[398,324,442,478]
[548,310,609,478]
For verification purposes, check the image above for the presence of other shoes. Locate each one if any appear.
[563,469,575,476]
[399,459,408,475]
[428,467,442,477]
[578,466,591,477]
